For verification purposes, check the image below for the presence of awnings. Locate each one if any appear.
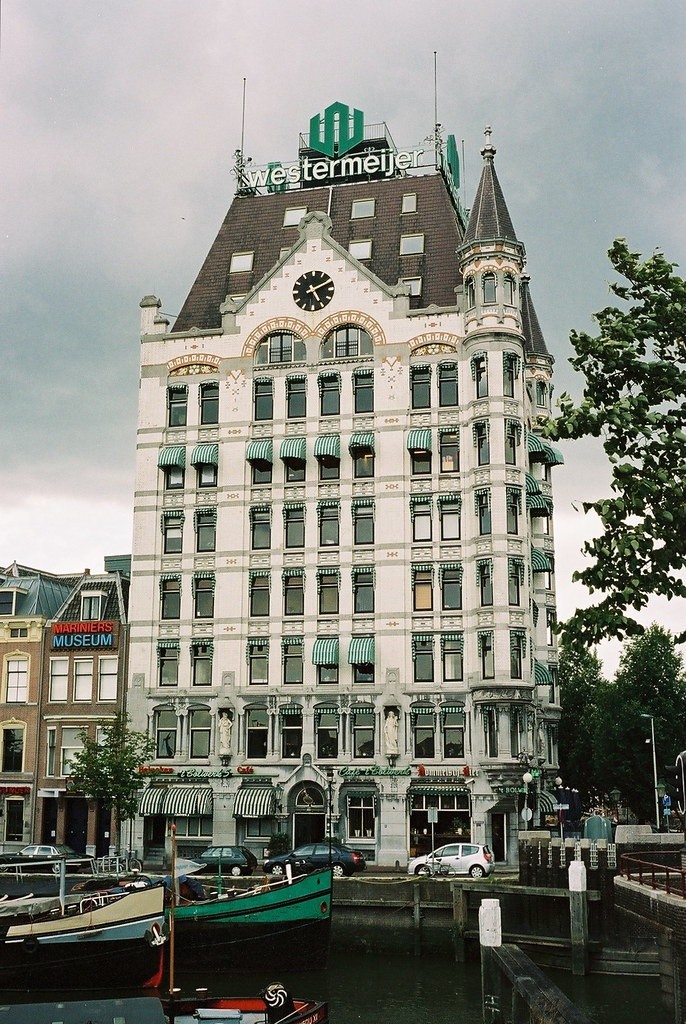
[540,791,557,813]
[166,352,555,406]
[463,269,524,299]
[157,550,552,599]
[159,473,554,534]
[139,787,277,820]
[157,635,555,685]
[158,418,564,468]
[337,786,379,818]
[405,783,473,814]
[279,704,534,733]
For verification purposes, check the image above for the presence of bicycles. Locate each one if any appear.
[104,844,143,874]
[418,854,457,878]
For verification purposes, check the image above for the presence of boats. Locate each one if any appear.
[0,877,167,969]
[162,861,334,923]
[0,987,330,1024]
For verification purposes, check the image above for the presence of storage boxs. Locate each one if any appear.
[194,1008,242,1024]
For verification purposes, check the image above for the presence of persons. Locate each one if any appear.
[217,713,233,754]
[383,712,400,755]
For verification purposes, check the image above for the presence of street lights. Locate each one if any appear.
[523,772,533,830]
[326,766,335,871]
[555,776,563,839]
[641,714,659,831]
[610,786,622,825]
[657,780,666,834]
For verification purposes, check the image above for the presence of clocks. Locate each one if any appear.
[293,271,334,311]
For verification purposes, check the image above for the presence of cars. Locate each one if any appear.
[0,843,94,874]
[263,841,367,878]
[408,842,495,879]
[186,845,258,877]
